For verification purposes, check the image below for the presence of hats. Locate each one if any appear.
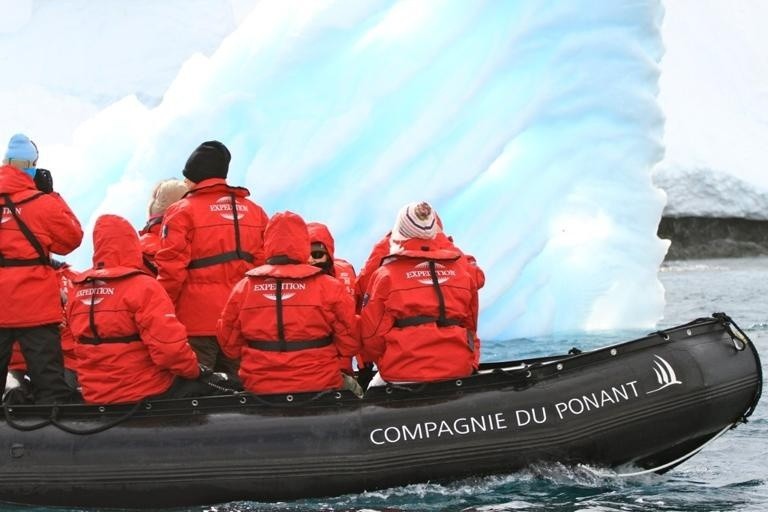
[391,201,438,240]
[183,141,230,182]
[4,134,38,177]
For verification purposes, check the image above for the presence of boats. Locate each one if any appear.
[0,307,766,512]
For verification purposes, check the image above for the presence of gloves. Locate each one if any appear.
[197,364,224,384]
[34,169,53,194]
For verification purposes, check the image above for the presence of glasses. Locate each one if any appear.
[310,250,327,258]
